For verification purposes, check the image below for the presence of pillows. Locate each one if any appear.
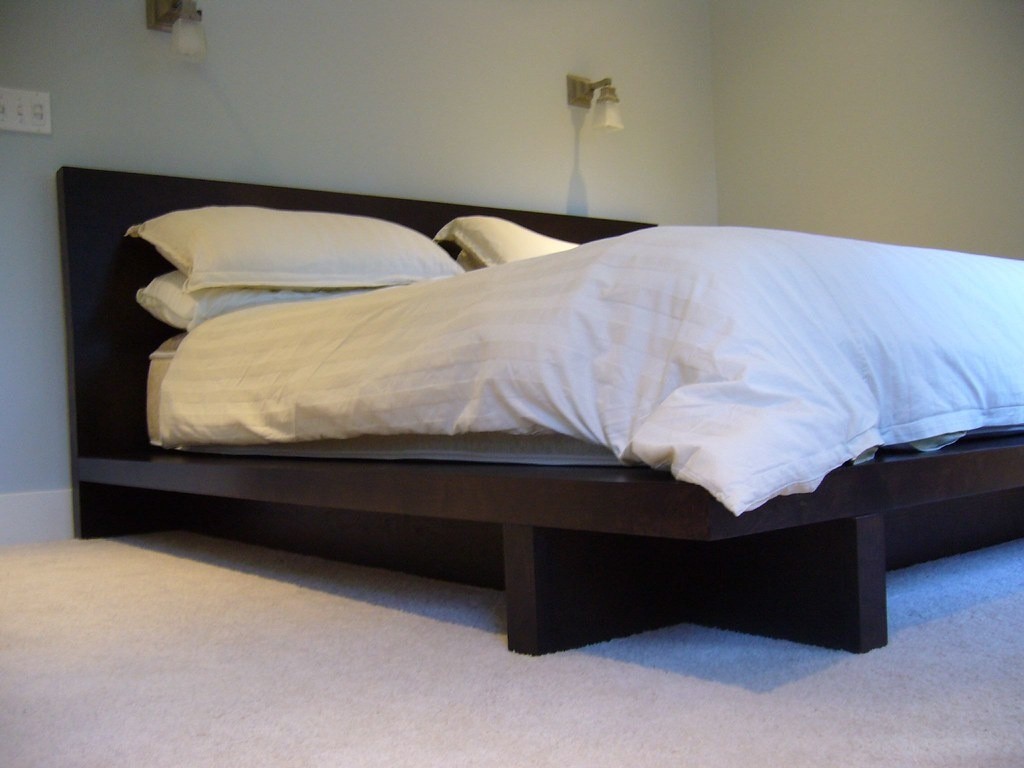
[434,215,579,269]
[124,203,467,291]
[456,248,481,272]
[136,270,372,334]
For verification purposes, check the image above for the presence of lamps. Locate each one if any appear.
[146,0,207,63]
[566,74,625,134]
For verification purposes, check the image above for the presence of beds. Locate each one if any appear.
[56,165,1024,655]
